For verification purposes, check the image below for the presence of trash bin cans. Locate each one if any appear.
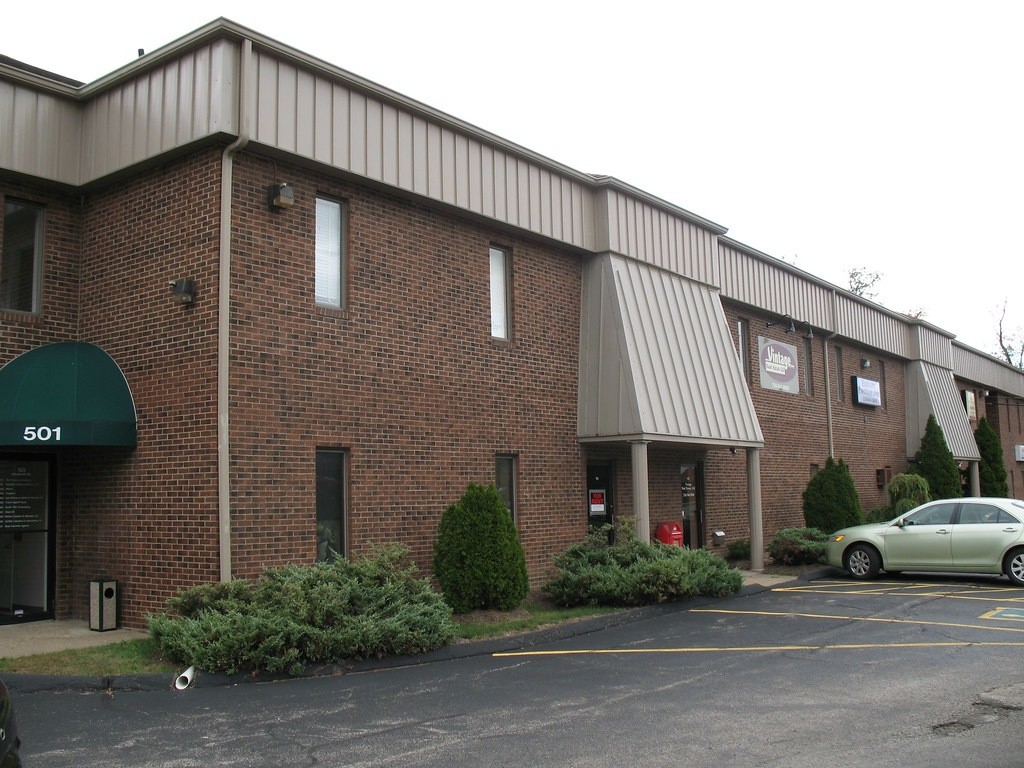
[656,521,683,549]
[87,575,121,630]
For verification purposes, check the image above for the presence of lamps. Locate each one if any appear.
[767,313,795,334]
[171,280,195,307]
[796,321,814,341]
[268,183,294,214]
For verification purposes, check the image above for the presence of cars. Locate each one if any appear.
[824,496,1024,582]
